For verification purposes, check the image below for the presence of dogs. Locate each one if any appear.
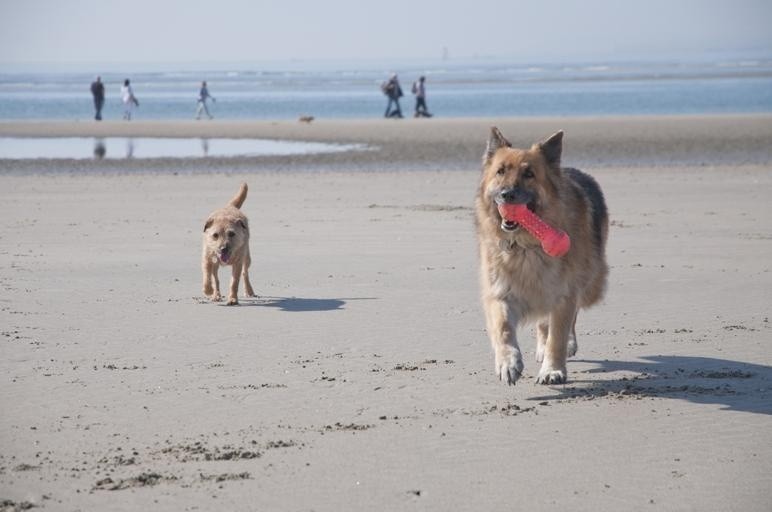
[472,125,610,386]
[201,181,257,306]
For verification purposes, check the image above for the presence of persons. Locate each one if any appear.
[90,76,106,120]
[196,137,217,157]
[413,75,434,119]
[124,136,136,159]
[94,137,106,161]
[120,78,137,121]
[195,80,217,120]
[382,73,406,119]
